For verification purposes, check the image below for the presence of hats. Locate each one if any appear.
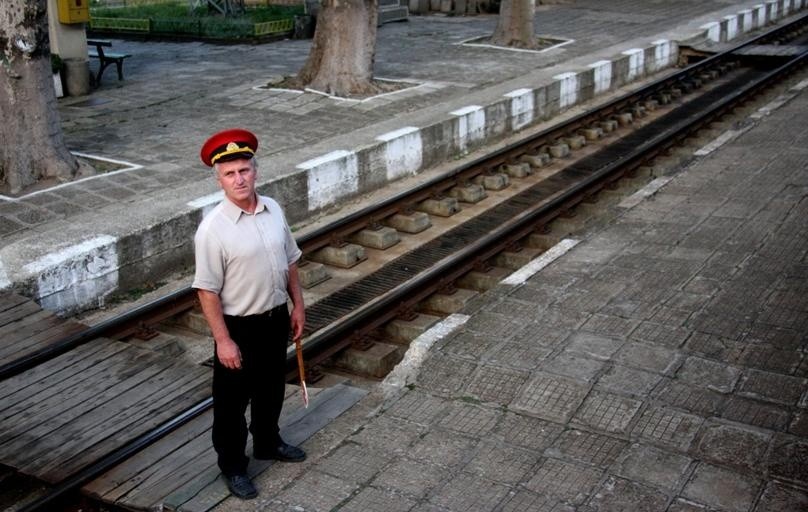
[200,128,259,168]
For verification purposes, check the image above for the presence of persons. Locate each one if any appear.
[191,127,308,501]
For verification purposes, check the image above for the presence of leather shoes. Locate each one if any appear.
[223,475,258,500]
[252,444,307,462]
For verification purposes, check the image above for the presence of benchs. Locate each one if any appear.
[86,40,131,86]
